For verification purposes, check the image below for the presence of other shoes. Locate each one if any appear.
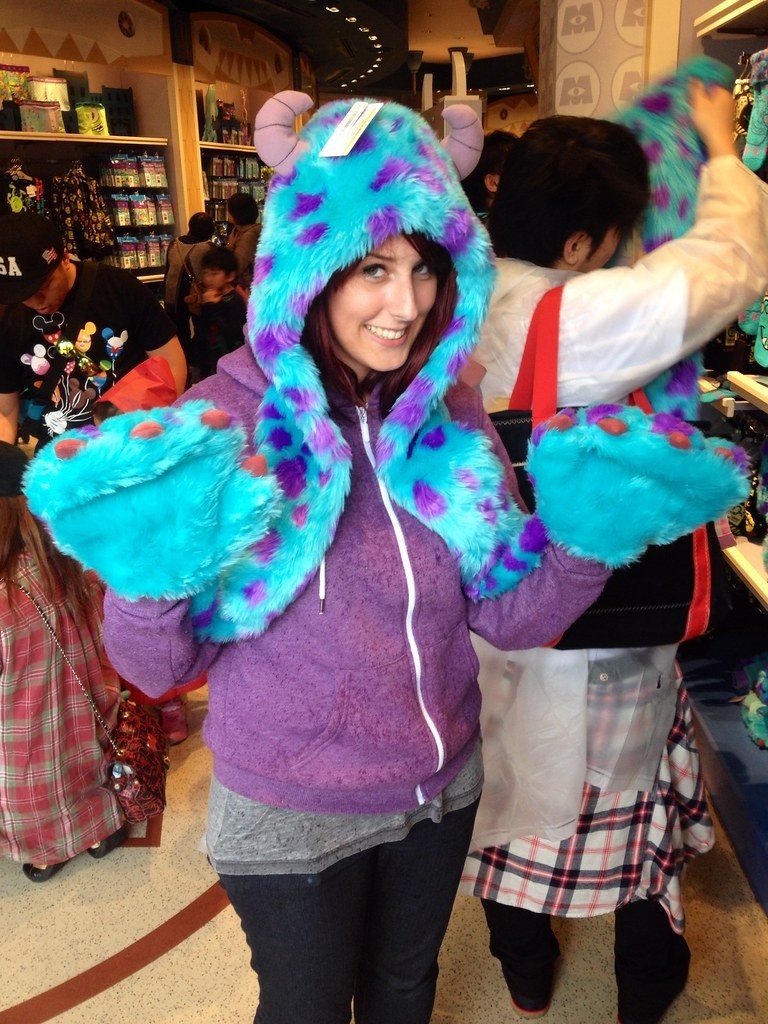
[510,986,551,1018]
[617,932,690,1024]
[22,858,70,883]
[88,823,130,859]
[160,698,187,746]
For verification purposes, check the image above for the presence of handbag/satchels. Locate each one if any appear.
[486,284,732,650]
[185,281,204,316]
[106,699,167,823]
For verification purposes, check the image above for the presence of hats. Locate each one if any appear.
[188,91,544,647]
[0,438,29,496]
[0,213,58,304]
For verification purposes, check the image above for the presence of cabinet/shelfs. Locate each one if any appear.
[687,0,768,920]
[0,52,303,283]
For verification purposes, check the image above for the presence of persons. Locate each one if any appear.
[23,78,768,1024]
[0,193,263,884]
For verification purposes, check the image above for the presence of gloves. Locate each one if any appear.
[523,400,754,569]
[23,400,285,603]
